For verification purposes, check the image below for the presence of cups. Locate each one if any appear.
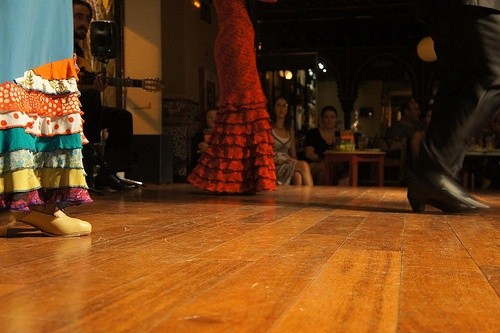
[359,135,367,150]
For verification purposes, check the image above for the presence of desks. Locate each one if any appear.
[324,150,386,187]
[464,152,500,192]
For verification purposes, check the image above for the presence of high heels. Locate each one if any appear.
[407,170,490,213]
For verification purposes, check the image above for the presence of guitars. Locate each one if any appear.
[76,56,161,93]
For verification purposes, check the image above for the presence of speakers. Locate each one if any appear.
[90,21,121,60]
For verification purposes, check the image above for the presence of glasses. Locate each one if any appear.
[276,103,289,107]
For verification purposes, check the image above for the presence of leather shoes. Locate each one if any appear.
[94,172,139,192]
[10,206,92,237]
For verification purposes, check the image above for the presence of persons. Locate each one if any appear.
[187,0,277,194]
[407,0,500,213]
[270,94,315,186]
[73,0,145,191]
[304,105,341,186]
[384,100,433,157]
[187,109,218,177]
[0,0,93,237]
